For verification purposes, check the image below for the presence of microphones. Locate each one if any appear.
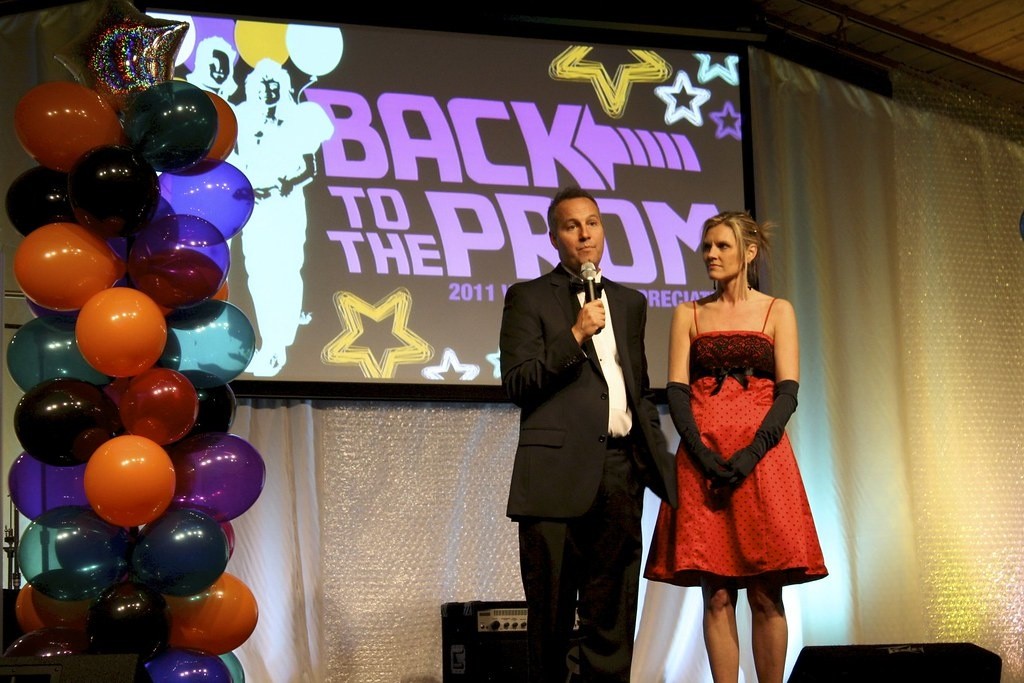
[580,260,597,303]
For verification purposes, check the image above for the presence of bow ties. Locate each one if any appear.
[565,272,604,298]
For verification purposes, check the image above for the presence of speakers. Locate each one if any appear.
[786,641,1004,682]
[440,601,580,682]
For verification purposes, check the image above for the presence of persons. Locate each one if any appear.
[499,188,680,683]
[642,211,829,683]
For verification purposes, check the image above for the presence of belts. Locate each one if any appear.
[606,437,631,451]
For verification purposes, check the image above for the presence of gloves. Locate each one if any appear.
[665,382,740,489]
[710,380,799,495]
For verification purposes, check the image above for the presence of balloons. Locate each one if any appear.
[4,0,266,683]
